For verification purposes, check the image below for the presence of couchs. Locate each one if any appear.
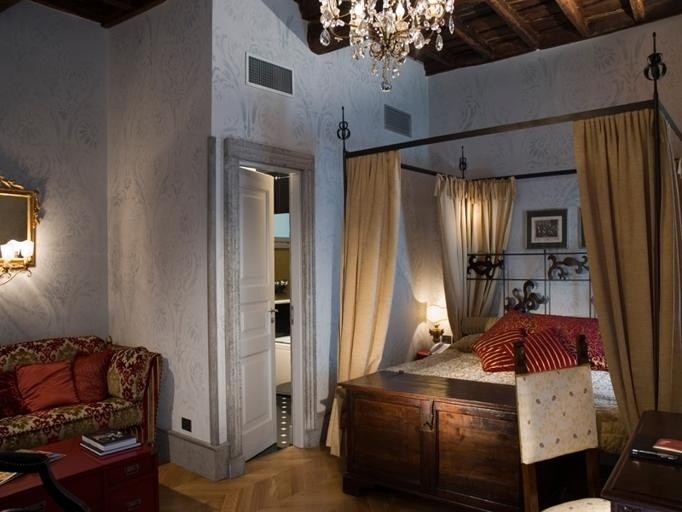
[0,335,162,450]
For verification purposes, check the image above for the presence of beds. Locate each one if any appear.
[380,247,627,459]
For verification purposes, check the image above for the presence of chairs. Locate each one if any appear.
[517,363,613,512]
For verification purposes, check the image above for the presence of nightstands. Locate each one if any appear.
[417,350,431,360]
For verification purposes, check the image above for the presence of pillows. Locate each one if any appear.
[504,326,578,373]
[471,327,530,372]
[586,329,609,370]
[72,351,110,403]
[15,360,81,411]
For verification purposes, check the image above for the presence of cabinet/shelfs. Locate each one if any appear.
[335,370,575,511]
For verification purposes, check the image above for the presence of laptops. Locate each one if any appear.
[630,410,682,465]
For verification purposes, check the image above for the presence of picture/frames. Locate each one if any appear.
[526,208,568,249]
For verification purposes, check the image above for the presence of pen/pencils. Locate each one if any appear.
[632,448,677,460]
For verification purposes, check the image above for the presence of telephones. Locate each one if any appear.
[429,341,451,354]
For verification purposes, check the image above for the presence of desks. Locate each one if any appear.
[601,408,682,512]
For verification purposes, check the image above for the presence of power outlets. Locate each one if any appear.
[181,417,192,432]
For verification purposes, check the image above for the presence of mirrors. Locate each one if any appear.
[0,175,42,270]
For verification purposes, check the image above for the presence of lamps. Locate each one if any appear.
[0,239,18,275]
[316,0,458,93]
[20,239,34,270]
[427,303,446,343]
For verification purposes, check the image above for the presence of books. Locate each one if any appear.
[0,449,66,485]
[79,441,141,456]
[653,437,682,453]
[82,430,135,449]
